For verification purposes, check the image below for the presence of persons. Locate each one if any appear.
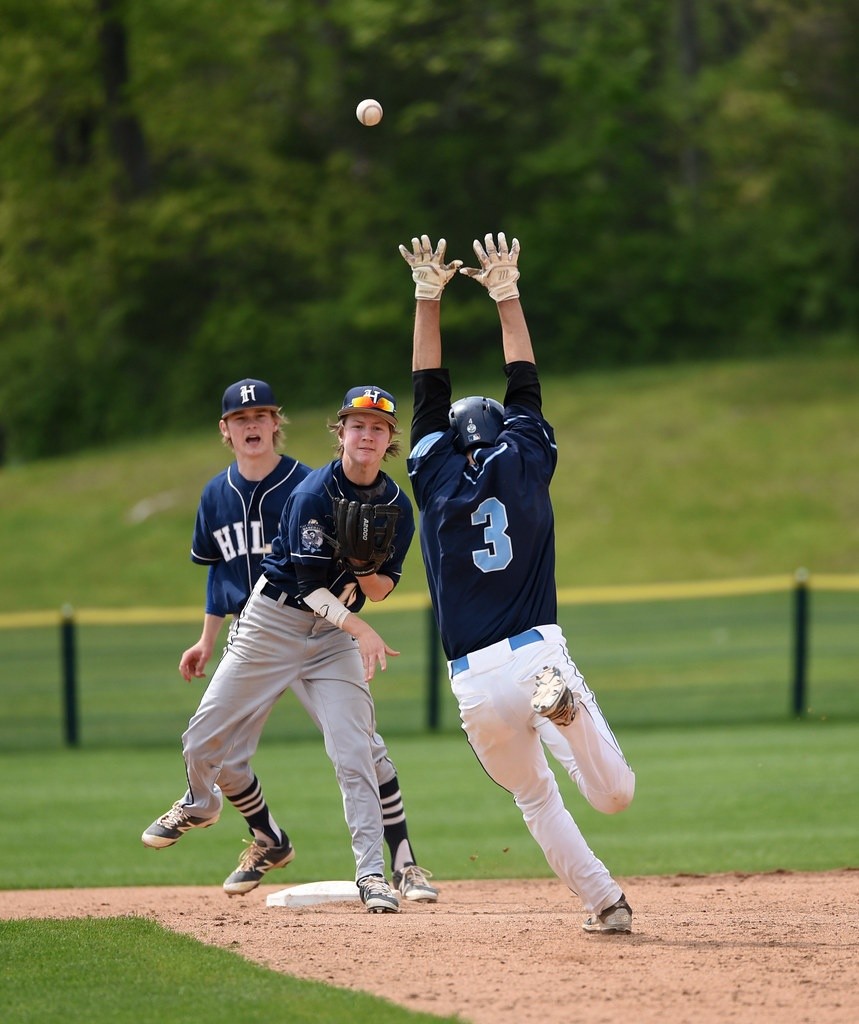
[140,386,399,912]
[396,232,635,934]
[179,380,438,904]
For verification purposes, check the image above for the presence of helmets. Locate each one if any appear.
[448,396,506,448]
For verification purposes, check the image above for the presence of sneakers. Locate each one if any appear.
[141,803,218,849]
[530,667,575,725]
[223,826,296,898]
[582,896,633,934]
[357,873,398,912]
[392,865,438,899]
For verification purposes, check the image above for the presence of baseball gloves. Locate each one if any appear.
[324,497,401,577]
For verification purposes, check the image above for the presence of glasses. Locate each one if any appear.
[345,395,397,415]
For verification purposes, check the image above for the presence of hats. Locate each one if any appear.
[221,378,279,419]
[336,385,398,427]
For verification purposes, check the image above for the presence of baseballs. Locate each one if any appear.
[355,98,384,127]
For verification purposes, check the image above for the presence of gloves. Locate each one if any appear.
[398,236,464,300]
[460,233,522,303]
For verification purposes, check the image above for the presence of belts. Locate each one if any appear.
[451,630,544,678]
[261,581,323,618]
